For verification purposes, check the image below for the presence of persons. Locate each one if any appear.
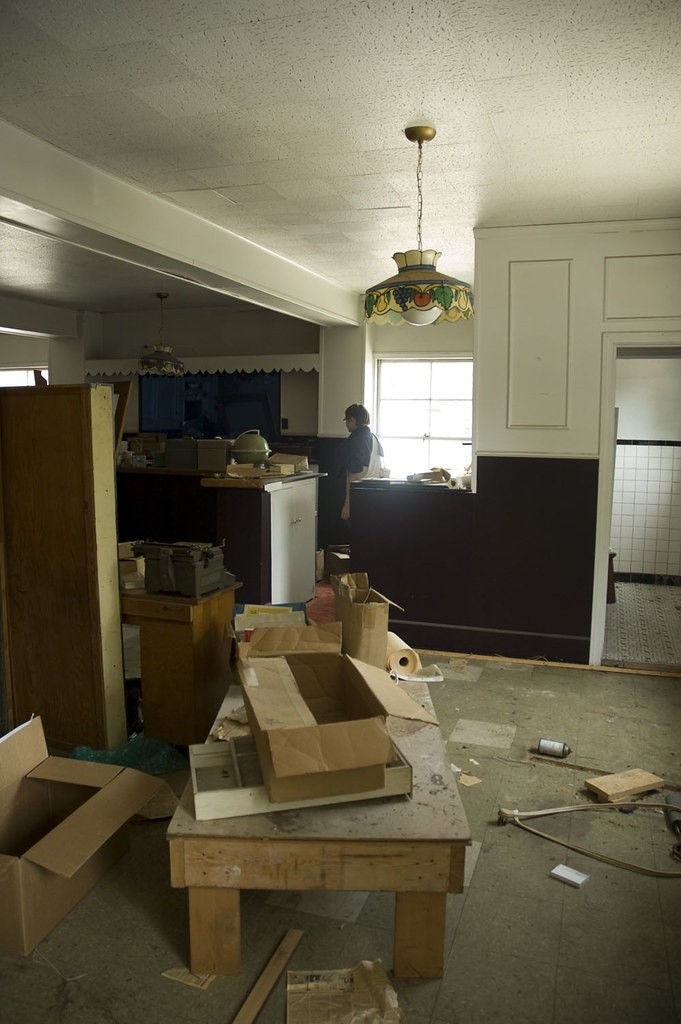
[340,404,387,519]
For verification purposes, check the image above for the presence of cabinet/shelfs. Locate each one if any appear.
[0,380,133,754]
[200,473,327,604]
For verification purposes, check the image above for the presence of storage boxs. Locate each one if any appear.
[234,572,404,803]
[144,550,224,595]
[315,548,326,583]
[127,433,167,457]
[328,544,350,575]
[0,712,182,957]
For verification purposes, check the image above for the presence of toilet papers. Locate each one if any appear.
[385,629,444,682]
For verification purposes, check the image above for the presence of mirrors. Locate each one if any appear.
[138,369,282,438]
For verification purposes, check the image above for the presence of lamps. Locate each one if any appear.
[364,121,476,328]
[131,293,184,380]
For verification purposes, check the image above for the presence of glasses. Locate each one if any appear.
[343,417,349,422]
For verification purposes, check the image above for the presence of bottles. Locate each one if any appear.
[537,738,573,758]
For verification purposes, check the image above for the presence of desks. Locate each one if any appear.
[165,663,473,979]
[120,581,245,748]
